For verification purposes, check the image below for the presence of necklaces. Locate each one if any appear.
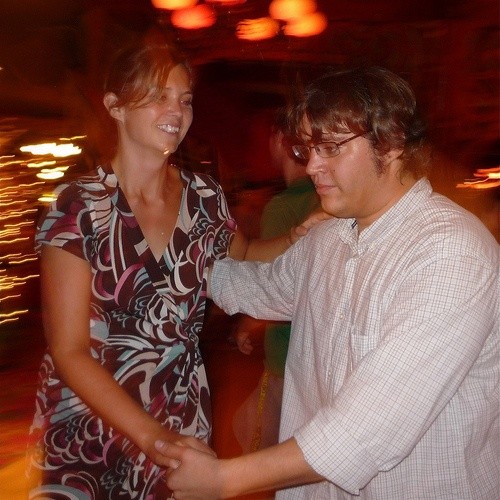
[136,217,176,237]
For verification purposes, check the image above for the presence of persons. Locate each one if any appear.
[236,107,325,448]
[24,44,335,500]
[154,66,500,500]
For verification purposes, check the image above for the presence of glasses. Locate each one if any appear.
[290,129,373,159]
